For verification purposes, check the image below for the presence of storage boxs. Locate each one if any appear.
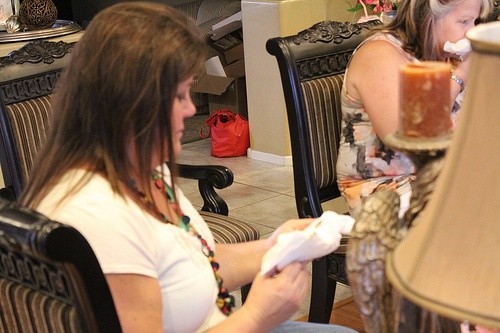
[193,56,249,120]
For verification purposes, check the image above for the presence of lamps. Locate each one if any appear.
[387,17,500,329]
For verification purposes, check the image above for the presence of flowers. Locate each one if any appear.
[347,0,396,15]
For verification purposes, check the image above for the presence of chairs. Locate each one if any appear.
[267,20,385,325]
[0,40,260,305]
[0,196,124,333]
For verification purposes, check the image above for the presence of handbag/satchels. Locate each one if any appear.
[199,110,249,158]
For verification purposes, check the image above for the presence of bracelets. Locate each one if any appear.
[451,74,465,92]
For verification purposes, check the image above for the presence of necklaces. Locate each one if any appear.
[118,168,235,317]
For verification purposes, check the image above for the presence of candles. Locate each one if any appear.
[401,61,451,140]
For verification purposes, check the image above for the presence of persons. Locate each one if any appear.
[336,0,496,216]
[17,2,359,333]
